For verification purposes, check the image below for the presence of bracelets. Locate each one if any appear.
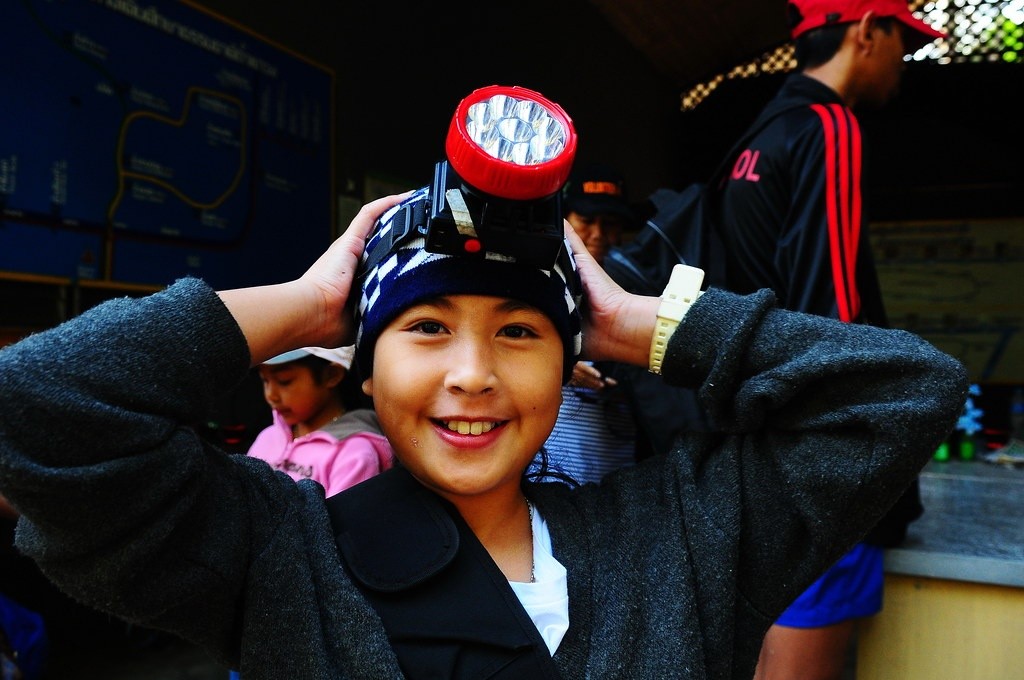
[648,262,707,375]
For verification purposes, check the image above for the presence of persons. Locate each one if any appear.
[524,171,633,486]
[0,188,971,680]
[245,345,394,498]
[695,0,923,680]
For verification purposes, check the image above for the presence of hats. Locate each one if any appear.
[354,182,584,384]
[261,346,356,371]
[565,171,657,218]
[789,0,948,56]
